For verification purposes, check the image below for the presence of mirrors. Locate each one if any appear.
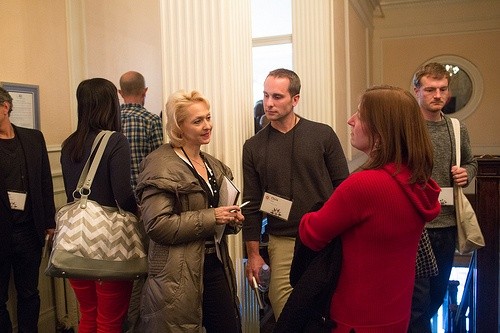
[411,54,485,120]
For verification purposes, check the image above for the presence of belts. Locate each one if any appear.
[204,247,216,254]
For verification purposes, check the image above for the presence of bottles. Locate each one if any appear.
[257,264,271,292]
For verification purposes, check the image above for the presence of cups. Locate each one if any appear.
[438,188,454,205]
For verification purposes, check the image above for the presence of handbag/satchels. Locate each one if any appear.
[450,117,486,255]
[44,130,149,280]
[414,228,439,281]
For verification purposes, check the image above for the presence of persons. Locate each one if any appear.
[243,70,351,323]
[132,87,245,333]
[118,71,164,333]
[405,63,478,333]
[300,85,441,333]
[60,78,141,333]
[0,85,56,333]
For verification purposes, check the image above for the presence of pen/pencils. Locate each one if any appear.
[228,201,251,213]
